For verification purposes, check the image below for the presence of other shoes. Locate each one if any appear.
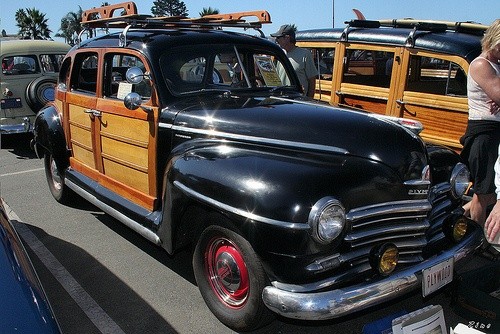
[475,243,500,263]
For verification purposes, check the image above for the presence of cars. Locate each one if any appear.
[31,1,483,334]
[0,40,73,135]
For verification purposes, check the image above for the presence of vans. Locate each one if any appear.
[271,9,491,157]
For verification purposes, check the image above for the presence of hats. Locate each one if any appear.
[271,24,296,40]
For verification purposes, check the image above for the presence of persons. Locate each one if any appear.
[269,24,320,104]
[484,143,500,247]
[459,18,500,231]
